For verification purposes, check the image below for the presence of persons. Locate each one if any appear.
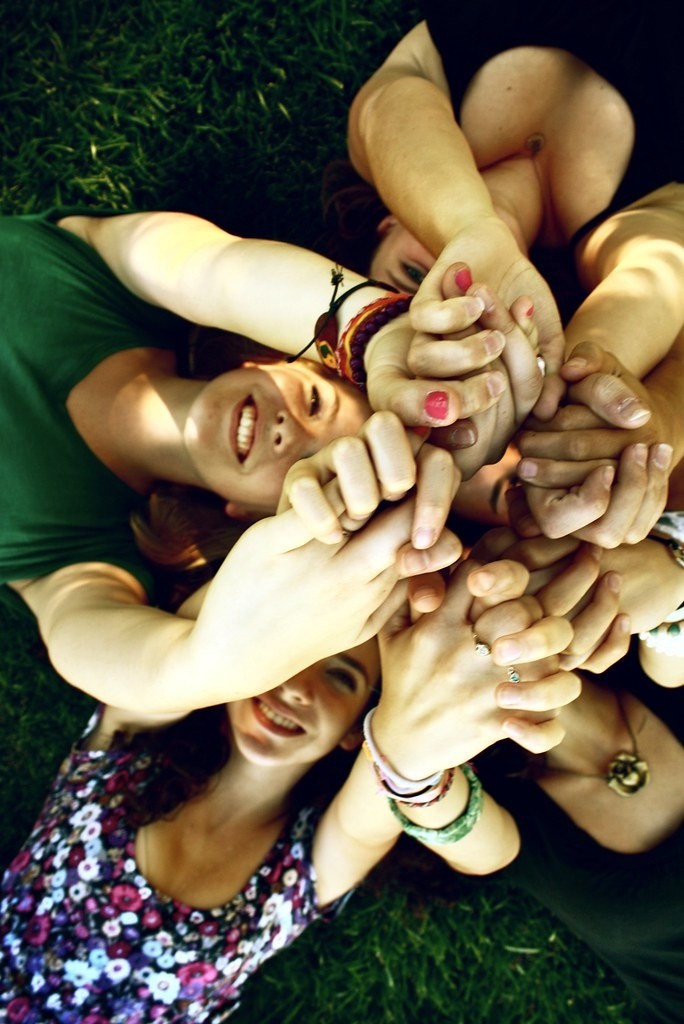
[0,1,684,1024]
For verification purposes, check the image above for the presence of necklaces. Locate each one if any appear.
[508,133,546,183]
[541,690,652,801]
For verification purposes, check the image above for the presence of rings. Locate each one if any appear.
[467,624,492,658]
[507,666,522,684]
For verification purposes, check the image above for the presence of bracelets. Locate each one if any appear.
[648,511,684,637]
[647,534,684,569]
[395,768,456,809]
[373,765,447,804]
[384,764,482,847]
[335,295,407,385]
[653,512,684,623]
[362,706,442,791]
[349,299,410,386]
[286,264,400,371]
[363,739,435,795]
